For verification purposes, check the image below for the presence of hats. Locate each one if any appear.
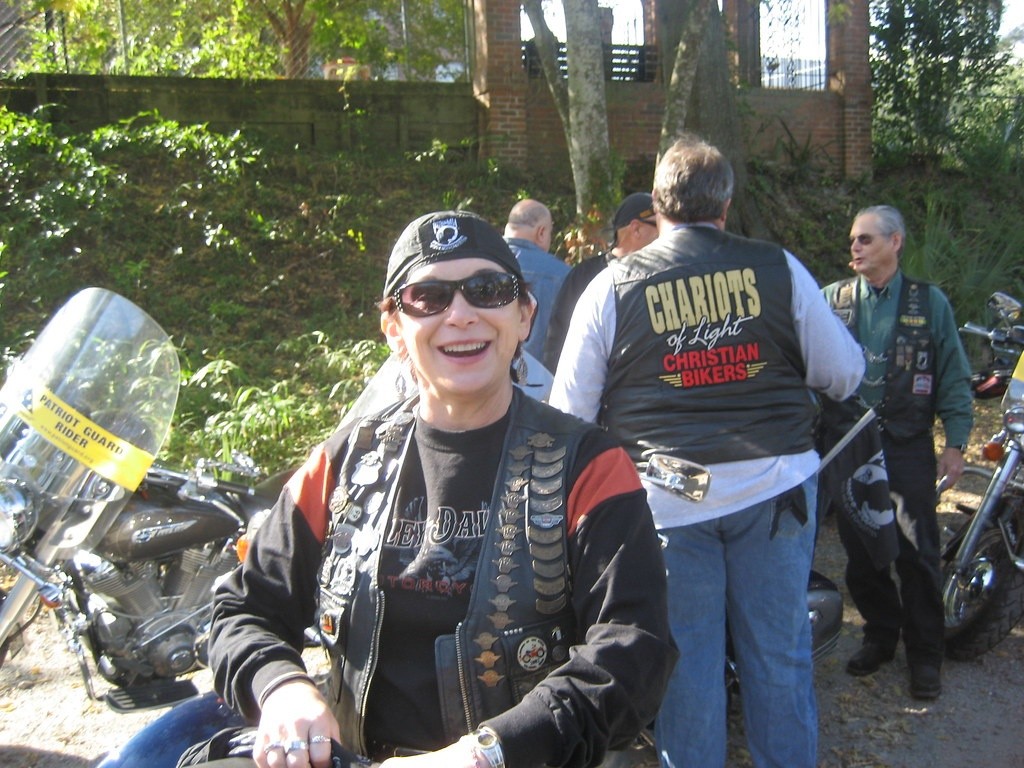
[382,211,523,307]
[613,191,656,231]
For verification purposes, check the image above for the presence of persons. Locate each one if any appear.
[549,136,867,768]
[95,211,681,768]
[502,197,572,363]
[542,192,659,377]
[820,204,975,698]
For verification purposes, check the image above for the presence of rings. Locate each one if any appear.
[264,742,284,754]
[284,739,309,753]
[309,735,330,743]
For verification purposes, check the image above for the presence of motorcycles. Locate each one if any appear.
[901,291,1024,664]
[0,286,310,716]
[173,563,846,768]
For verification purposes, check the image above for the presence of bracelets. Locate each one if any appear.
[460,734,480,768]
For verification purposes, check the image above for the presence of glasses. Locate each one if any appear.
[393,272,520,317]
[848,231,888,248]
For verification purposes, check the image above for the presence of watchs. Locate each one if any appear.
[951,443,967,454]
[470,728,506,768]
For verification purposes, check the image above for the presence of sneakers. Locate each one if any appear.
[906,648,941,698]
[846,640,894,675]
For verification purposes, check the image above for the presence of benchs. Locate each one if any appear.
[523,41,659,83]
